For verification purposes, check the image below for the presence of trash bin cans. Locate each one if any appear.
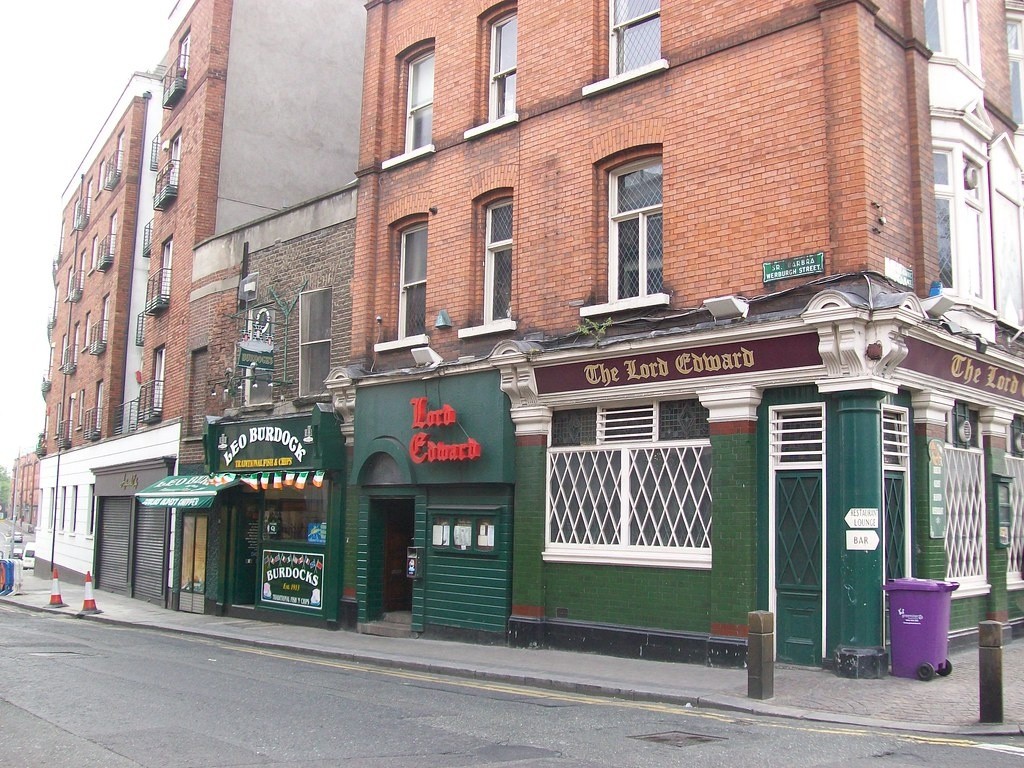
[883,577,961,682]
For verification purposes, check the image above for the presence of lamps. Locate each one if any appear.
[250,376,259,387]
[410,346,445,367]
[960,334,988,353]
[224,381,229,393]
[268,375,274,388]
[210,384,218,395]
[238,379,243,389]
[703,295,750,322]
[925,318,962,334]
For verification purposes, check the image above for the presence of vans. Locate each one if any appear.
[22,542,35,570]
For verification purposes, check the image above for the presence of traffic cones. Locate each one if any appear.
[77,569,105,617]
[42,567,70,608]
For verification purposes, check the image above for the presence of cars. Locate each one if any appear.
[14,531,24,543]
[9,548,23,559]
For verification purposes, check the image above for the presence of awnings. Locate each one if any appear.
[135,474,241,508]
